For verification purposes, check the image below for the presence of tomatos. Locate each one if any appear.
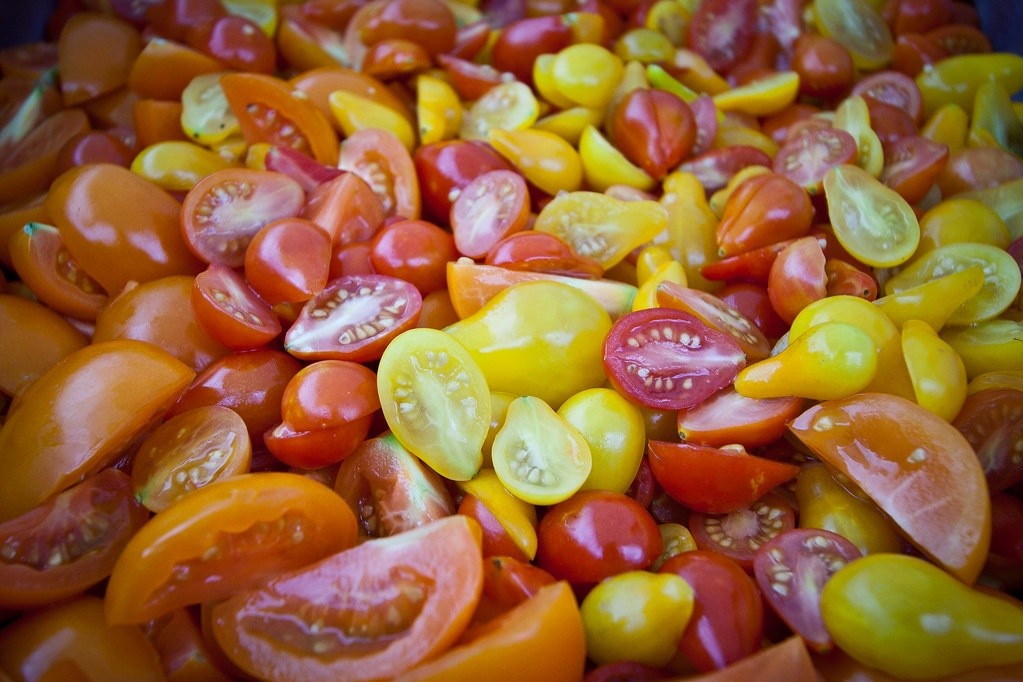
[0,0,1023,682]
